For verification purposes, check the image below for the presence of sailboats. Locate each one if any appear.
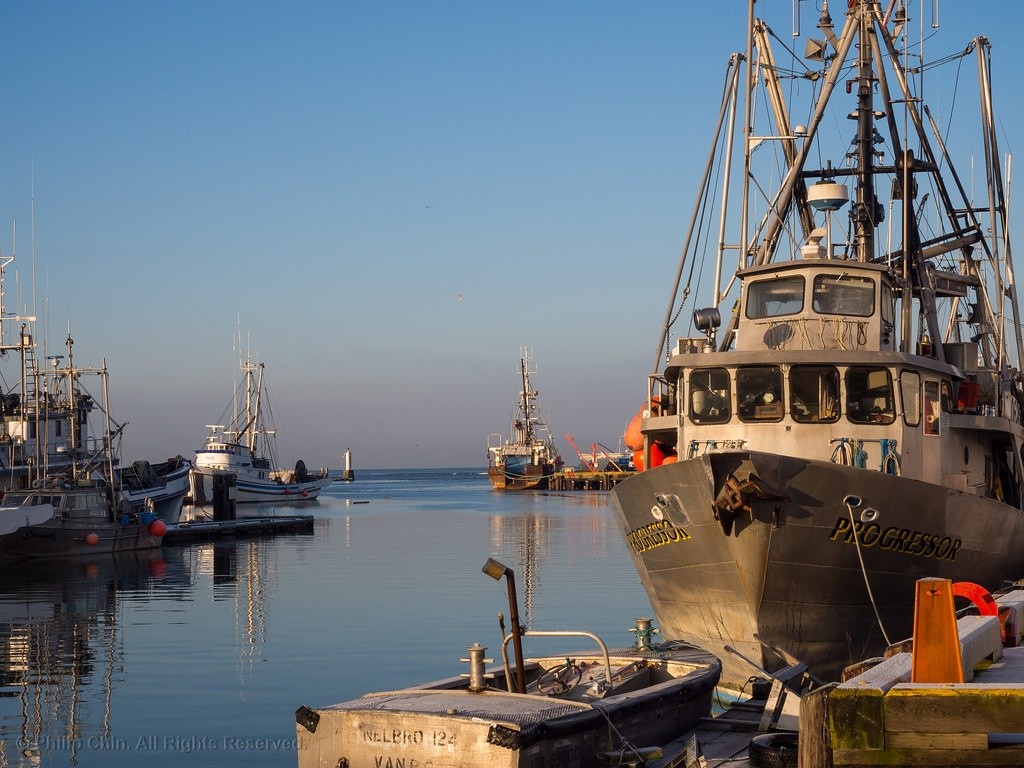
[608,1,1023,695]
[0,158,194,558]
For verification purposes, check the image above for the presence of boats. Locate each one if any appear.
[488,345,564,490]
[297,555,722,768]
[190,311,334,504]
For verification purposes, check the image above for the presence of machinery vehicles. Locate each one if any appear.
[564,430,598,472]
